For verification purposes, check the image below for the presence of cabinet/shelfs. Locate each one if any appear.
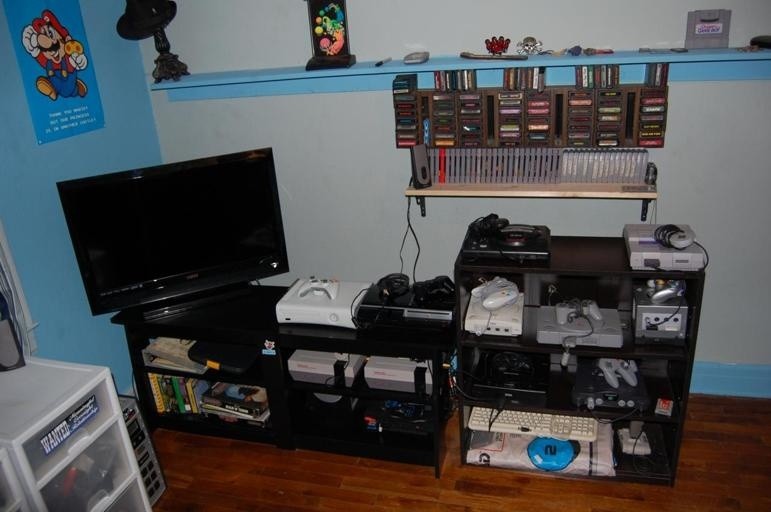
[0,356,155,512]
[110,325,462,480]
[455,272,709,487]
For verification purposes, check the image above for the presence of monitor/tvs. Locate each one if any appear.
[56,147,290,322]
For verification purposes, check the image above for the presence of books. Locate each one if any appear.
[147,372,272,430]
[141,336,212,374]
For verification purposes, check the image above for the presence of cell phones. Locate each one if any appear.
[403,51,430,64]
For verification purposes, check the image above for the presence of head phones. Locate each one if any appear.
[377,274,409,300]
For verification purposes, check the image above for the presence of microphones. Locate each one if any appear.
[351,288,368,328]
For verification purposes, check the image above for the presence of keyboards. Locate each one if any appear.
[468,406,598,441]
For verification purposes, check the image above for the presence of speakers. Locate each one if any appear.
[0,292,26,371]
[410,144,432,188]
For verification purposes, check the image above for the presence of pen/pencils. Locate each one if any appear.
[375,56,392,68]
